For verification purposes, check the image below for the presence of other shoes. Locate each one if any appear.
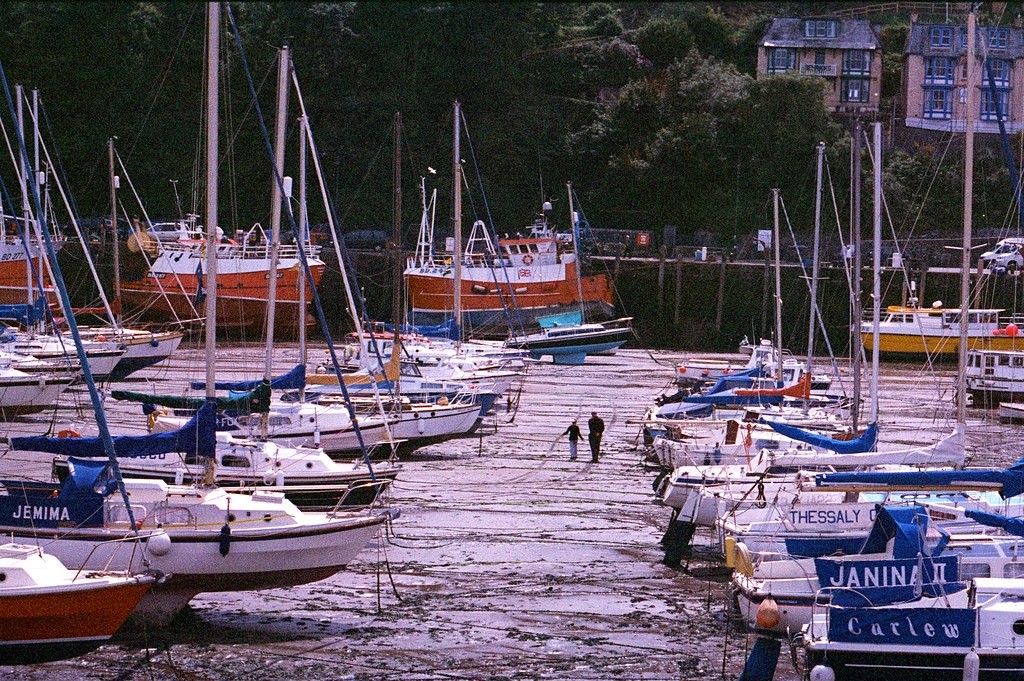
[591,459,599,463]
[570,457,578,459]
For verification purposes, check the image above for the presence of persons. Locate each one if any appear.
[588,411,604,462]
[562,419,585,459]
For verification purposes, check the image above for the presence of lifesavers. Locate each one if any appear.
[522,254,535,265]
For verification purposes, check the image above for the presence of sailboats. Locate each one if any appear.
[144,114,483,463]
[303,100,534,435]
[0,1,402,634]
[639,10,1024,636]
[51,44,402,507]
[0,68,166,667]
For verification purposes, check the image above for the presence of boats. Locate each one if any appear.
[953,349,1024,408]
[0,201,66,305]
[403,177,615,340]
[790,553,1024,681]
[503,316,635,364]
[113,213,324,342]
[848,282,1024,363]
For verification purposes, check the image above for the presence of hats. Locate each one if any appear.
[572,419,576,422]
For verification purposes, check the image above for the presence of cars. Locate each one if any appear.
[146,222,192,242]
[996,246,1023,270]
[979,238,1024,268]
[328,230,390,252]
